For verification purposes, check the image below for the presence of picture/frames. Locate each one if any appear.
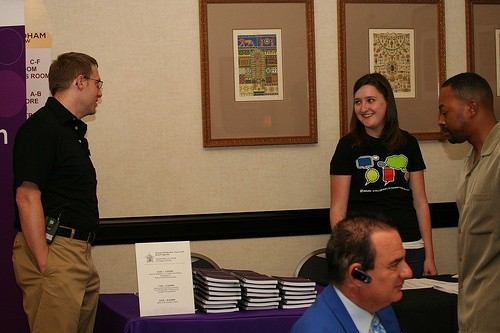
[197,0,318,148]
[338,1,447,139]
[464,0,500,123]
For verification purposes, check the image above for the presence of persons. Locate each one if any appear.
[329,73,438,278]
[289,211,412,333]
[12,52,103,333]
[438,72,500,333]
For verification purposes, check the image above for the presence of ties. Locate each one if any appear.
[372,315,386,333]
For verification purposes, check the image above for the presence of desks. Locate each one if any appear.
[94,273,459,333]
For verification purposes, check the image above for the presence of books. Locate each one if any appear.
[192,267,317,314]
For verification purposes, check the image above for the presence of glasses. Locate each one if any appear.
[76,76,103,89]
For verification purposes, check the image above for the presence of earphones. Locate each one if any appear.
[351,268,370,283]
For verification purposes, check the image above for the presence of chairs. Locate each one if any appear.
[294,248,333,287]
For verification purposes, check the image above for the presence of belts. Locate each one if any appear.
[55,227,95,246]
